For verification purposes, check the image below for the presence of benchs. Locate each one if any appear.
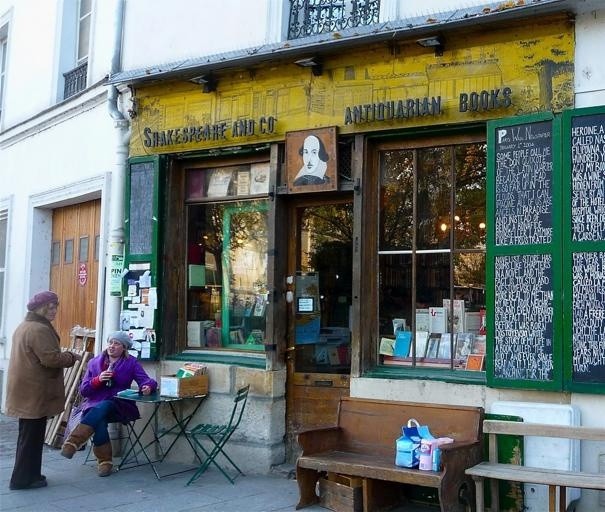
[295,395,485,512]
[464,419,605,512]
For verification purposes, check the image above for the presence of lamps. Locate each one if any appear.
[186,73,220,93]
[293,56,324,76]
[416,35,448,56]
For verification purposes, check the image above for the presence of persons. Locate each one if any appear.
[5,291,82,491]
[61,330,158,477]
[292,133,331,187]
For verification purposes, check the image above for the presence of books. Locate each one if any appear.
[189,234,266,348]
[187,163,269,201]
[175,362,208,379]
[379,299,487,372]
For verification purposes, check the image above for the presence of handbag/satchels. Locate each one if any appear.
[394,418,436,468]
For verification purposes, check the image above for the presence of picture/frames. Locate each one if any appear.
[285,125,340,195]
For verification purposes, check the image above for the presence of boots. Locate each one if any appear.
[94,440,113,477]
[61,422,95,459]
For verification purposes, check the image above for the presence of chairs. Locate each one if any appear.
[185,383,251,485]
[83,416,143,465]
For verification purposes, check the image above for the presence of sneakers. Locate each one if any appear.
[10,475,47,489]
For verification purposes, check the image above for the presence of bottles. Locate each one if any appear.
[215,309,221,328]
[106,361,114,387]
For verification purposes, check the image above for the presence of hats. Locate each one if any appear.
[27,292,58,310]
[107,331,133,349]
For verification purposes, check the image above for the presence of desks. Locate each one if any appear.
[113,389,210,481]
